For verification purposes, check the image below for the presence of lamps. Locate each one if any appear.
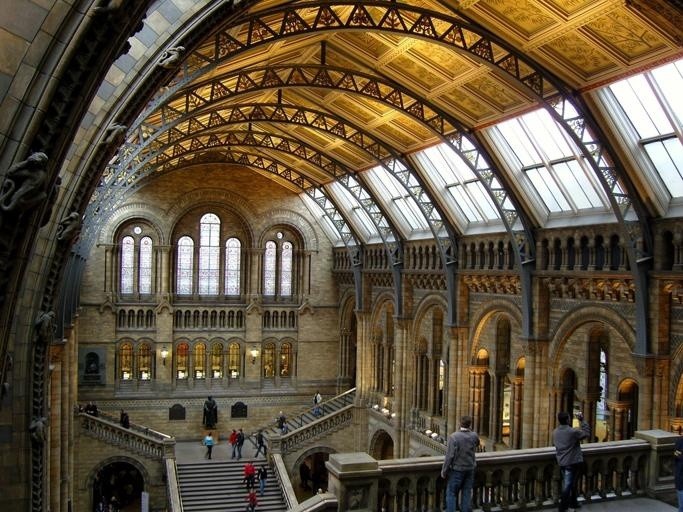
[250,346,259,365]
[159,345,169,367]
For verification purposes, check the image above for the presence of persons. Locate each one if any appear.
[280,422,287,435]
[246,460,255,493]
[298,461,310,488]
[256,464,267,496]
[203,393,217,430]
[274,409,285,433]
[235,427,244,459]
[243,487,258,512]
[251,428,266,459]
[203,431,213,459]
[77,397,98,416]
[312,389,322,415]
[95,494,120,512]
[671,421,682,511]
[439,416,481,512]
[227,428,237,459]
[240,460,250,485]
[118,407,128,428]
[552,409,589,512]
